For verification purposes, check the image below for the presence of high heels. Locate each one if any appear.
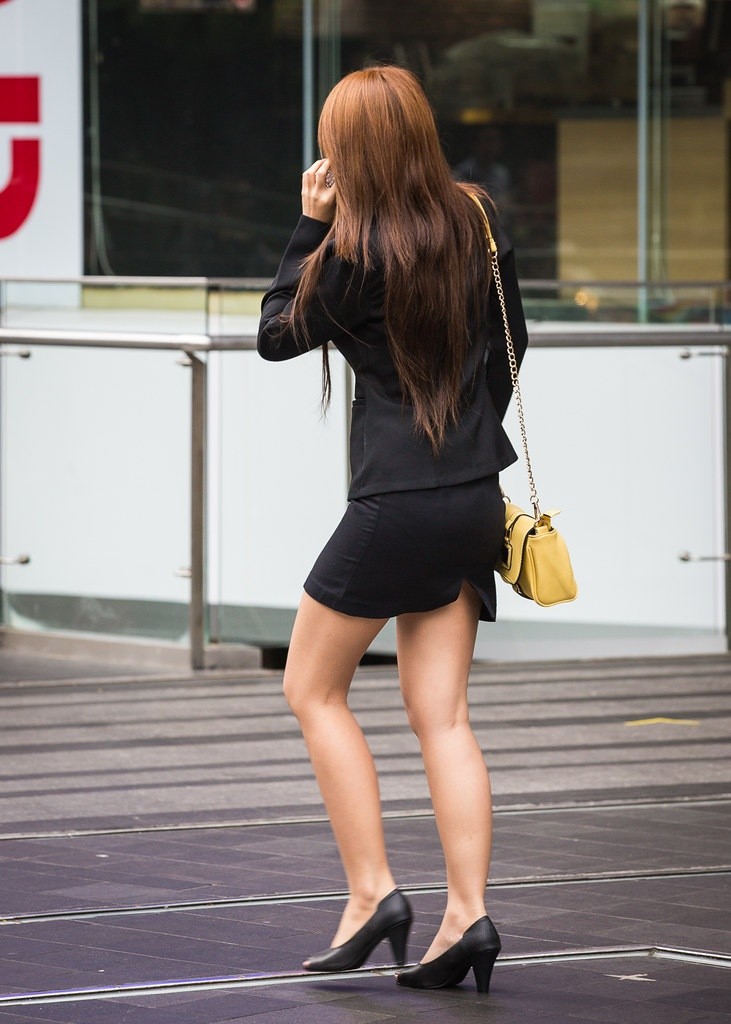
[302,889,414,973]
[394,916,501,994]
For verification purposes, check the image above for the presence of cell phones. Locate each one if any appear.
[326,167,336,188]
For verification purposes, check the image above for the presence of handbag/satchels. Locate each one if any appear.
[497,495,580,607]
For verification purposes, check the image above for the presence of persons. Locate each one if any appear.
[257,64,529,1000]
